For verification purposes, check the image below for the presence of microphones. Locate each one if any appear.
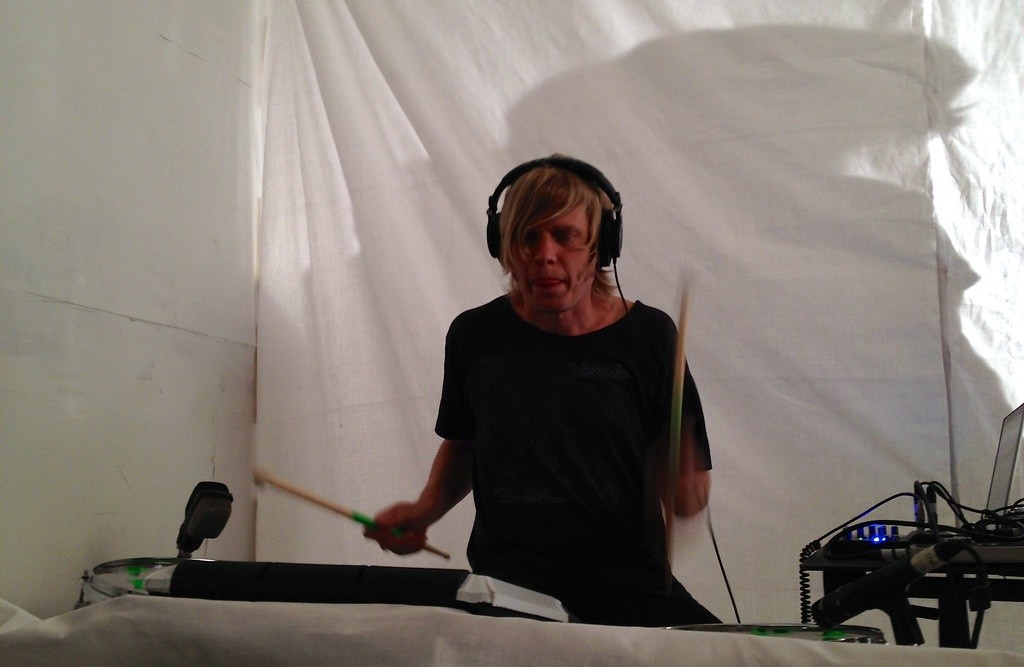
[177,482,235,553]
[811,538,962,629]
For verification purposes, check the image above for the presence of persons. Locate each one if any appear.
[363,152,725,628]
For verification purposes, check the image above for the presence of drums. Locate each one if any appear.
[72,555,219,609]
[664,624,887,646]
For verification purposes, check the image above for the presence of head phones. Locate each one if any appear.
[487,155,623,268]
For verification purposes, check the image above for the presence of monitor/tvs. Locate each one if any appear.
[984,402,1024,531]
